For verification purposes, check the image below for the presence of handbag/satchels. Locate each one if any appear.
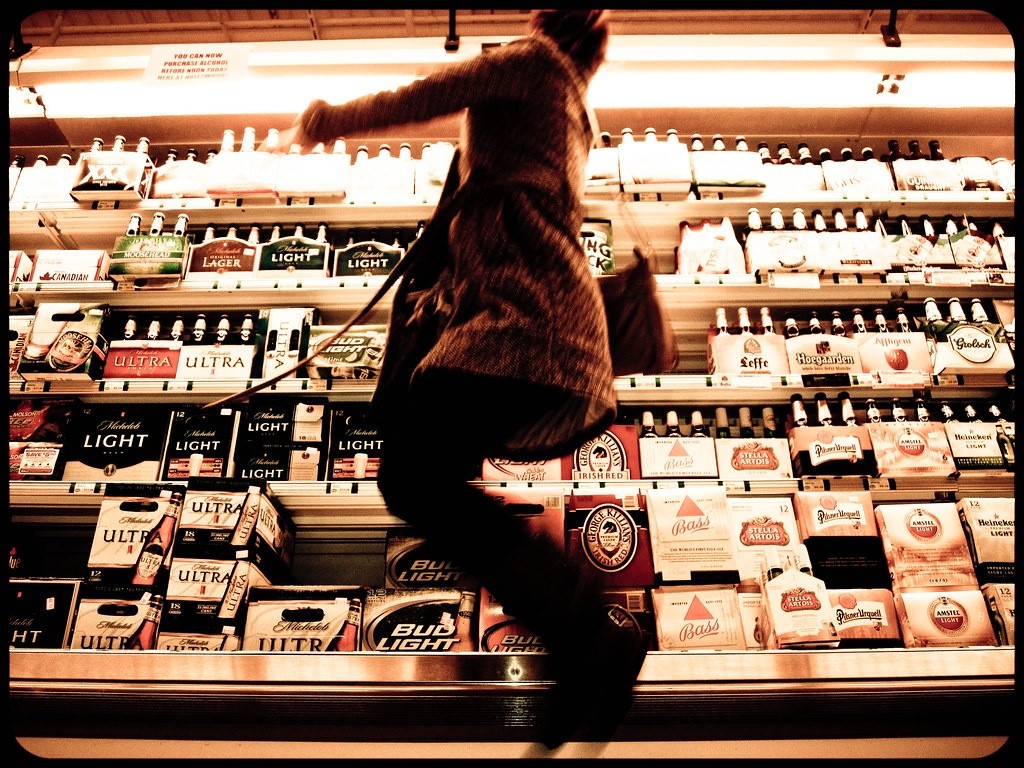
[596,249,679,375]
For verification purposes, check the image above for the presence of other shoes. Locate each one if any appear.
[531,627,645,751]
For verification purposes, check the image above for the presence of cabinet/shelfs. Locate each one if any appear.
[4,134,1019,727]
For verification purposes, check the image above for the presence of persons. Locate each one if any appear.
[301,9,647,750]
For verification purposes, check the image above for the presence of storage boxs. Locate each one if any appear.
[7,142,1021,655]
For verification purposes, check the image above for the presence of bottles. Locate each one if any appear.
[9,126,1014,647]
[9,125,1014,438]
